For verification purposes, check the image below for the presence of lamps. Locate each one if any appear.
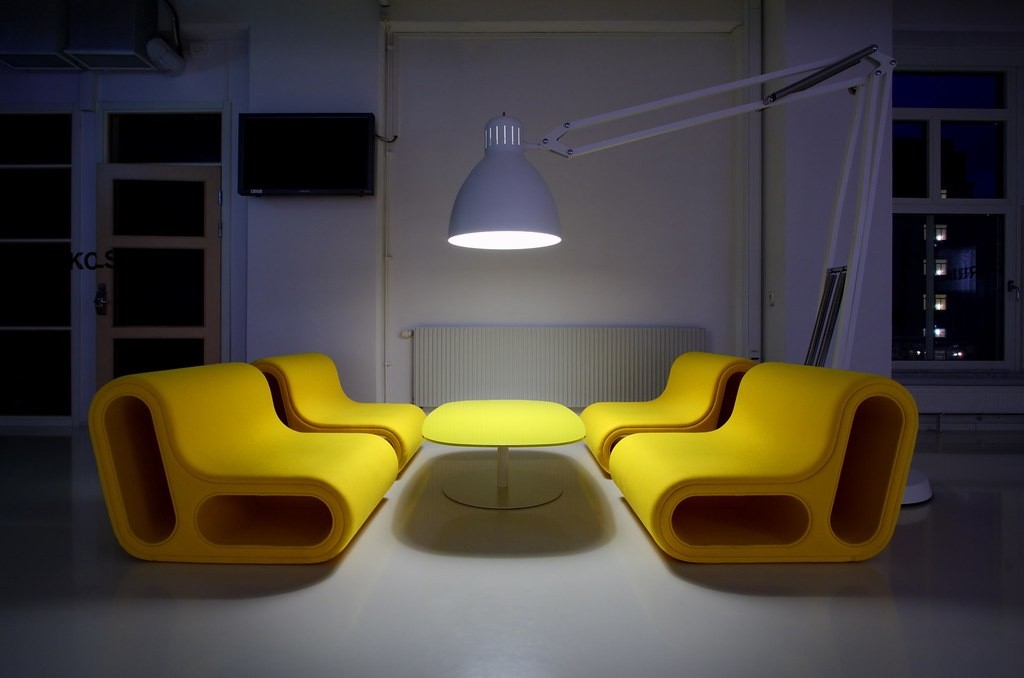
[446,44,934,508]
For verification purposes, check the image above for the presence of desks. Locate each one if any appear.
[422,399,586,510]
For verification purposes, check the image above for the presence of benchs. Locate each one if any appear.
[87,361,400,565]
[578,351,756,475]
[251,352,427,479]
[608,361,920,565]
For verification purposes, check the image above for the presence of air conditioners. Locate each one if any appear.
[0,0,161,72]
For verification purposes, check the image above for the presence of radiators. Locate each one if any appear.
[402,325,705,409]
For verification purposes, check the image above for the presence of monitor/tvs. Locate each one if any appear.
[238,113,375,197]
